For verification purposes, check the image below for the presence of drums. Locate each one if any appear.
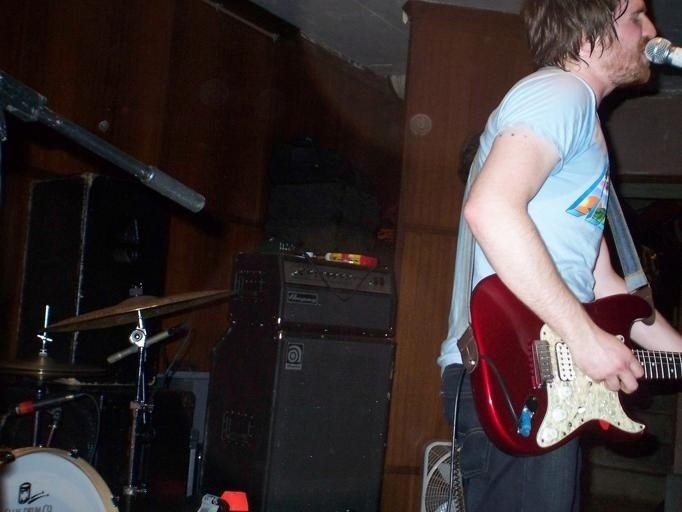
[0,446,120,512]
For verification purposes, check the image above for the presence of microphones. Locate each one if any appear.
[15,393,85,415]
[645,37,682,68]
[106,328,175,364]
[38,110,207,213]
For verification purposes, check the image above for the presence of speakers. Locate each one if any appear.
[196,335,396,512]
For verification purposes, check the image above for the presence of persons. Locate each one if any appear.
[438,0,682,511]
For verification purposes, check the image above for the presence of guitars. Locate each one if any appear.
[466,273,682,456]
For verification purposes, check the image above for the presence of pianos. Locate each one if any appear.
[23,376,156,396]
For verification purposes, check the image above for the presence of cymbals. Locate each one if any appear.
[42,286,235,332]
[1,358,81,378]
